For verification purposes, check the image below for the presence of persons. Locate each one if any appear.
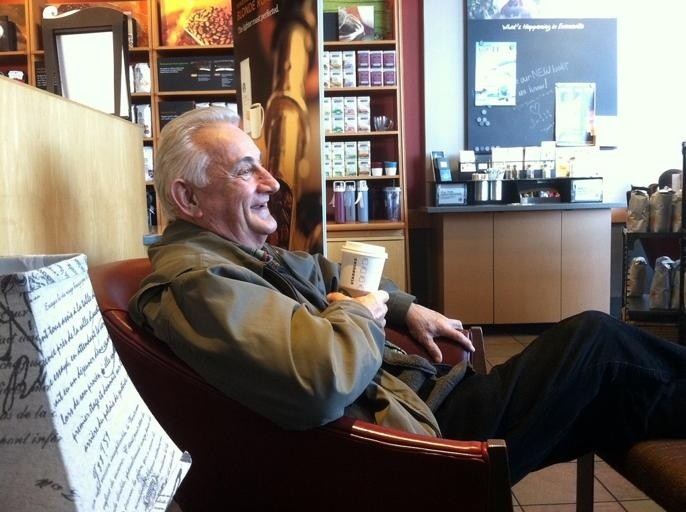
[127,105,685,487]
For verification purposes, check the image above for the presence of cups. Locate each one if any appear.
[371,163,384,177]
[337,239,389,303]
[249,102,265,141]
[384,185,402,223]
[373,114,394,132]
[384,159,398,177]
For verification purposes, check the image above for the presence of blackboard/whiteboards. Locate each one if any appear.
[463,17,618,155]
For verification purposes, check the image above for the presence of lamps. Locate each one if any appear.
[0,254,194,512]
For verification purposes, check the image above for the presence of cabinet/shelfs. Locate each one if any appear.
[419,203,618,324]
[622,169,685,346]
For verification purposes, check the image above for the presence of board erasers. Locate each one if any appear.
[599,145,616,150]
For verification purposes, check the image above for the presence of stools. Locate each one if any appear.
[576,436,685,512]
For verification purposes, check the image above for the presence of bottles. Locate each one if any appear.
[542,164,551,178]
[505,165,518,179]
[526,165,534,179]
[344,180,356,224]
[333,179,347,224]
[357,179,370,223]
